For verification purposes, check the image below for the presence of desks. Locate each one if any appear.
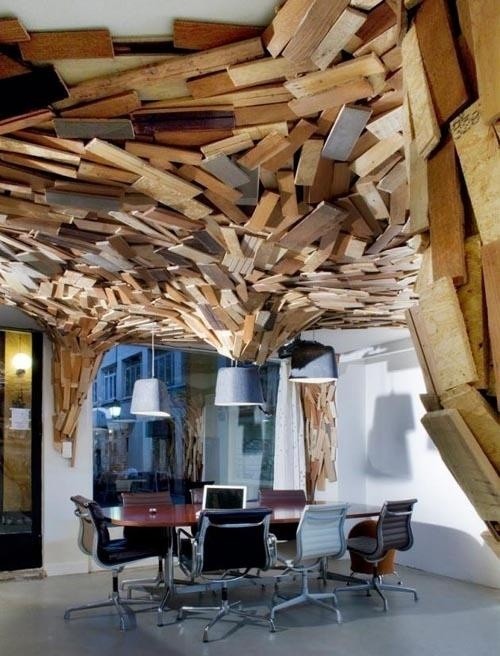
[97,501,390,627]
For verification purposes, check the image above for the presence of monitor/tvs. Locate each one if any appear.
[202,484,247,511]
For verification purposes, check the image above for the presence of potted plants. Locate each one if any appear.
[9,388,32,430]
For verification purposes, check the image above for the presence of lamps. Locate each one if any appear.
[287,328,336,383]
[130,332,171,418]
[14,353,29,376]
[215,356,264,406]
[108,345,121,416]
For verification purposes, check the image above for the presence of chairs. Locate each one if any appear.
[121,490,199,598]
[176,509,277,643]
[271,504,349,625]
[258,490,306,503]
[62,496,169,632]
[336,499,420,611]
[190,487,203,540]
[347,521,402,584]
